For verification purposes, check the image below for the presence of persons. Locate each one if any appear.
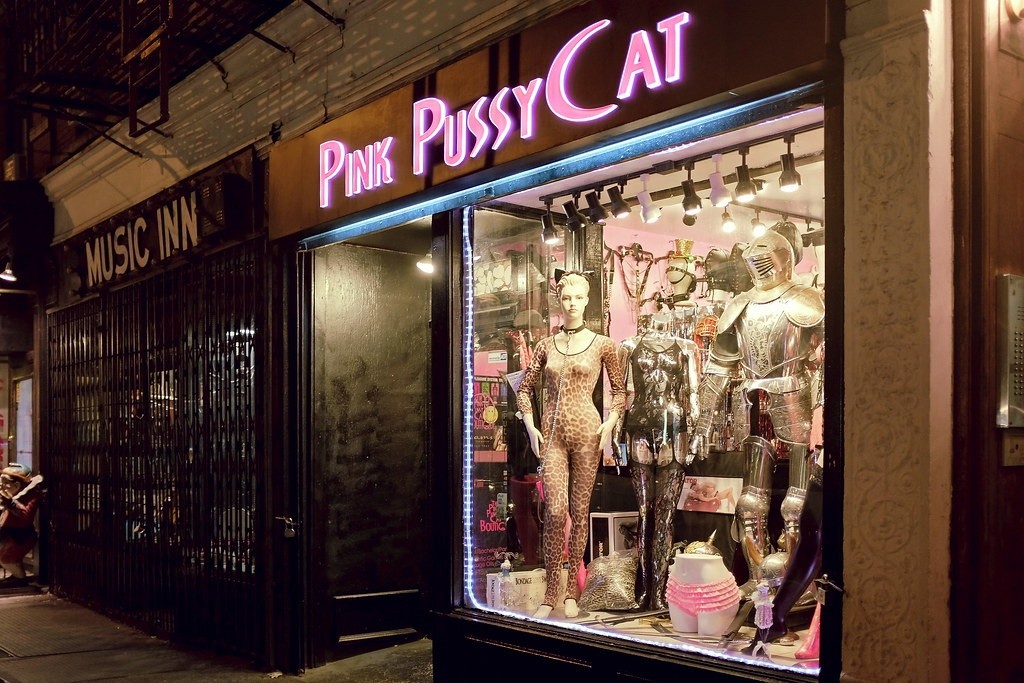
[738,449,826,656]
[0,462,43,588]
[517,273,626,620]
[668,296,696,339]
[727,241,755,298]
[662,238,698,312]
[616,311,698,617]
[768,222,805,284]
[704,242,731,303]
[685,228,826,603]
[689,481,736,512]
[665,553,739,637]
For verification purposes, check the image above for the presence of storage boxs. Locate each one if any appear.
[590,511,640,557]
[484,564,568,613]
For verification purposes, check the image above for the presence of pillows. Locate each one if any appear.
[583,555,639,611]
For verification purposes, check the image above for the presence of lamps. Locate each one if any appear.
[679,161,702,216]
[562,191,589,232]
[541,198,559,244]
[750,208,766,237]
[733,145,756,204]
[801,219,815,248]
[706,153,731,208]
[682,213,697,226]
[585,185,609,225]
[0,262,17,282]
[607,177,632,219]
[635,174,661,226]
[415,247,434,274]
[777,134,801,193]
[720,205,736,234]
